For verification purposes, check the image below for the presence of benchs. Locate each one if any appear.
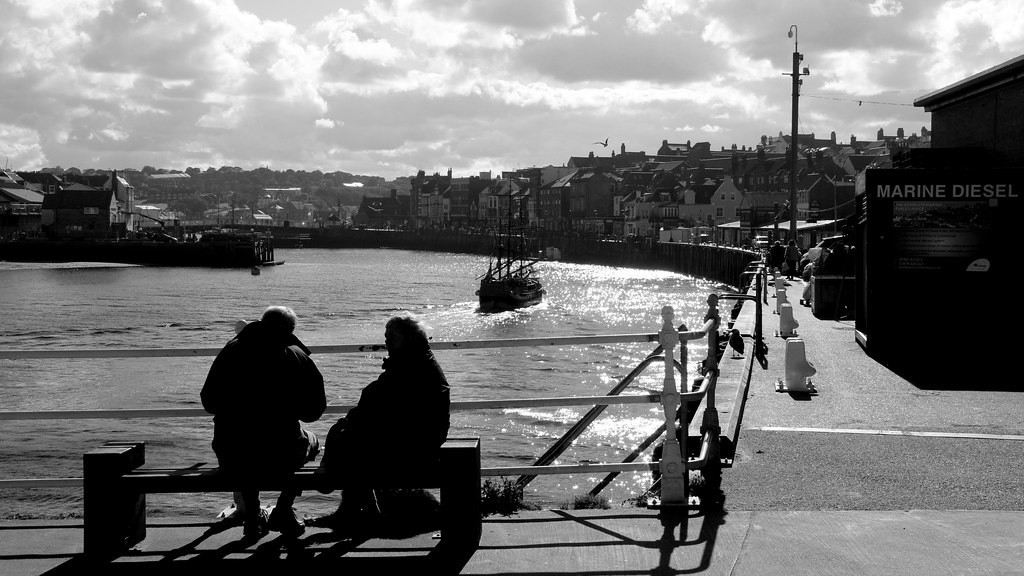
[81,434,483,552]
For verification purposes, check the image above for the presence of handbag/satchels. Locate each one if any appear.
[323,419,355,472]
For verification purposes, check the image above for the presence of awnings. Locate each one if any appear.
[716,218,846,231]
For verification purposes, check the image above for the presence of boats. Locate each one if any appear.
[1,185,279,270]
[475,174,546,310]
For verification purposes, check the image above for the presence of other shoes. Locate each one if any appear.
[242,510,269,537]
[267,507,305,534]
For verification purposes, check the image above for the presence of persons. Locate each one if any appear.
[198,304,331,540]
[769,238,799,281]
[744,237,749,249]
[304,314,452,528]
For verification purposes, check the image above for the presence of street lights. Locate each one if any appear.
[787,24,803,241]
